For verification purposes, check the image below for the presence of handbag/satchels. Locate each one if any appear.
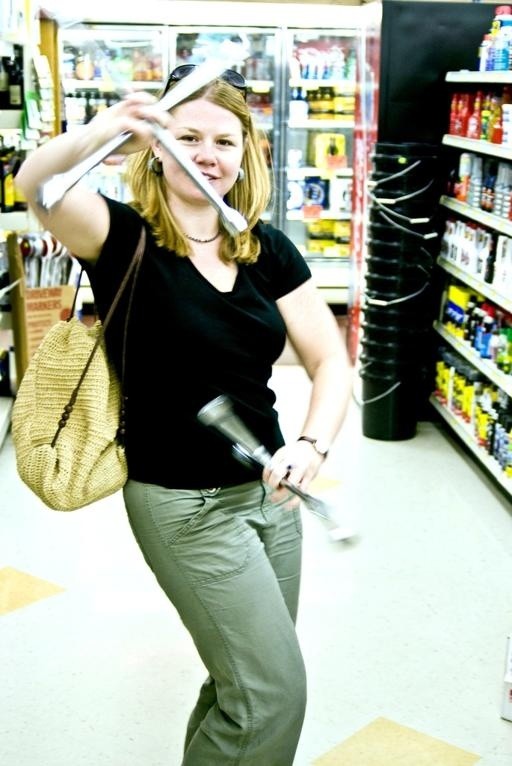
[11,210,149,514]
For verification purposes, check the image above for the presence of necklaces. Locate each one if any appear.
[182,229,222,243]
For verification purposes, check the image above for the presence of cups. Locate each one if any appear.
[287,148,302,168]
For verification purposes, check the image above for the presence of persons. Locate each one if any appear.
[16,63,352,766]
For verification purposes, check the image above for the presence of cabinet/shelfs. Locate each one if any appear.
[425,69,511,504]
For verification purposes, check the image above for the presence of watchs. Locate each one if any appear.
[297,437,330,462]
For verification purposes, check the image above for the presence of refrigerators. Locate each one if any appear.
[53,28,353,307]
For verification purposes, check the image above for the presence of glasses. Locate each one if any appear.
[161,63,249,104]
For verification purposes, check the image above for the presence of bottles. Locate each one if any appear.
[0,55,23,106]
[65,90,117,125]
[448,88,512,145]
[290,88,335,121]
[440,219,511,296]
[433,346,512,479]
[464,302,511,374]
[0,151,25,212]
[478,7,512,71]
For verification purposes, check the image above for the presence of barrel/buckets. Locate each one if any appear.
[348,142,440,441]
